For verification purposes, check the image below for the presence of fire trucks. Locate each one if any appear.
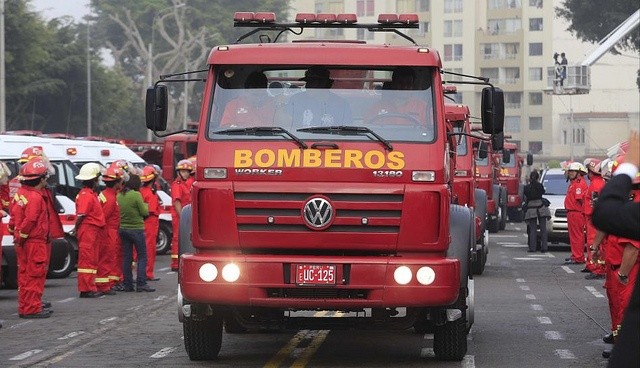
[475,115,510,232]
[1,130,198,184]
[145,13,503,360]
[502,137,533,222]
[444,86,502,275]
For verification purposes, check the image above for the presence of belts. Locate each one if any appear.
[611,265,621,271]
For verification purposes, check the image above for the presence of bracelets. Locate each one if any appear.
[615,269,631,284]
[590,244,599,251]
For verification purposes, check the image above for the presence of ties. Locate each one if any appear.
[80,291,99,298]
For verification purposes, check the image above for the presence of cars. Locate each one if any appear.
[537,168,586,248]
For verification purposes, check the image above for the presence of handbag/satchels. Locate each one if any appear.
[530,184,551,207]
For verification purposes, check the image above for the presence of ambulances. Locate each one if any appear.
[0,160,101,277]
[1,134,173,277]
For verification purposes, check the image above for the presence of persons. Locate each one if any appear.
[137,166,154,283]
[67,162,107,300]
[560,52,568,85]
[11,145,54,316]
[601,155,640,358]
[170,159,192,272]
[567,161,589,265]
[121,173,157,294]
[97,167,128,294]
[280,64,349,131]
[561,160,570,219]
[362,69,433,141]
[152,162,163,191]
[187,157,203,188]
[219,71,278,135]
[586,156,602,272]
[589,128,640,367]
[523,170,551,253]
[8,160,53,320]
[601,155,620,185]
[0,162,13,336]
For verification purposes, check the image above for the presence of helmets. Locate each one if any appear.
[560,161,571,171]
[149,164,161,172]
[611,155,640,185]
[140,165,158,183]
[15,156,48,181]
[102,166,130,182]
[110,159,129,171]
[176,159,193,170]
[568,162,588,175]
[583,158,602,175]
[17,146,44,163]
[75,162,101,181]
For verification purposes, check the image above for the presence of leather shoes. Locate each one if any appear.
[580,268,591,272]
[112,284,124,291]
[42,299,51,308]
[584,272,605,280]
[103,291,116,295]
[137,283,156,293]
[125,286,135,292]
[565,260,582,264]
[24,310,50,319]
[602,350,613,358]
[565,256,572,261]
[603,333,614,344]
[147,276,160,281]
[99,291,102,296]
